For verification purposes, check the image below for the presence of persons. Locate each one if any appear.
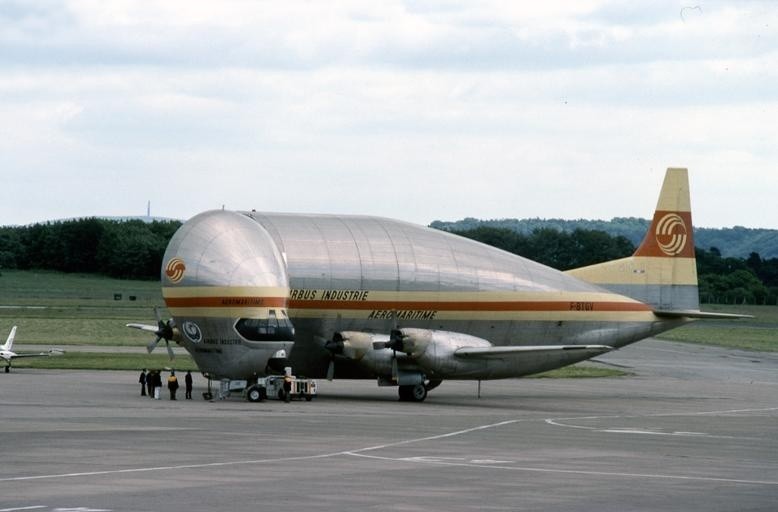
[284,376,291,402]
[139,368,147,396]
[150,369,157,398]
[145,369,153,396]
[168,369,179,400]
[154,370,162,399]
[185,370,193,399]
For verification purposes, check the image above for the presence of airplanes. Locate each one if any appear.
[125,168,755,401]
[0,325,49,373]
